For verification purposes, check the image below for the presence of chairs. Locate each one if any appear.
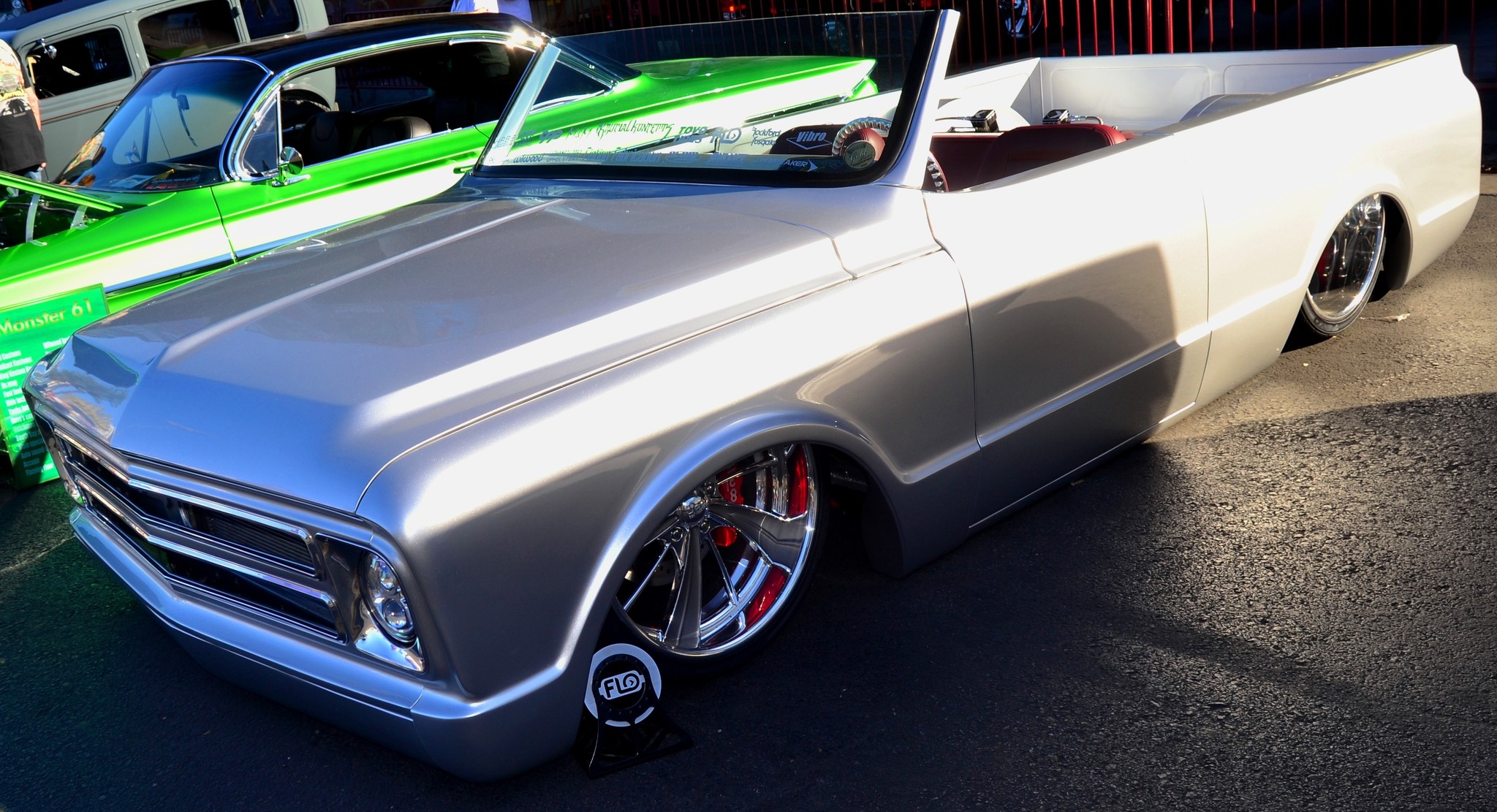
[365,115,438,153]
[980,118,1137,191]
[295,108,360,169]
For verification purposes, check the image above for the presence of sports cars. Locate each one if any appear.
[0,13,877,328]
[21,9,1478,779]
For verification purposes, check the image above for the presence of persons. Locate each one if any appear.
[0,37,48,197]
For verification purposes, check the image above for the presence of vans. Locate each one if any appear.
[9,0,541,185]
